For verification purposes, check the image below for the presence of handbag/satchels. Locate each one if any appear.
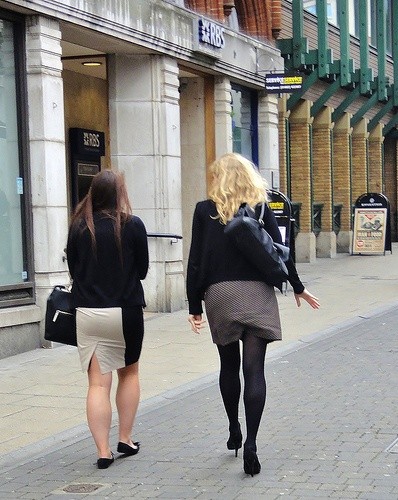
[43,280,78,349]
[224,200,291,294]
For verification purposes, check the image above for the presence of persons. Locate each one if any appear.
[67,168,149,470]
[185,152,320,479]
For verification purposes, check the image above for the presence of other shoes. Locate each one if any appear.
[117,441,140,455]
[96,452,115,470]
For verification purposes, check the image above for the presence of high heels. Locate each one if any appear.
[226,431,242,458]
[243,450,261,479]
